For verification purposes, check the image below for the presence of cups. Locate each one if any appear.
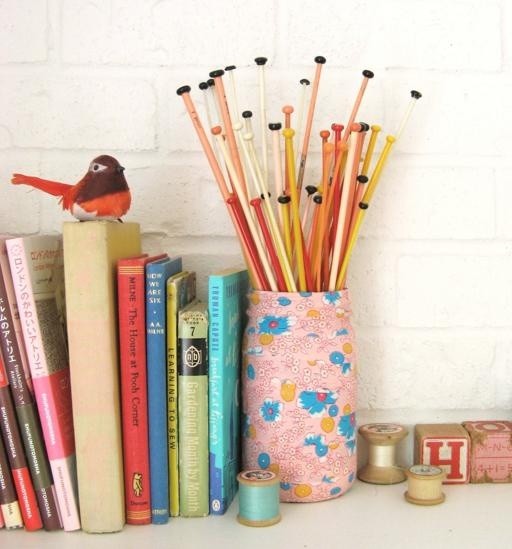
[238,287,360,505]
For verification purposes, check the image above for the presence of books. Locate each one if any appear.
[1,217,253,536]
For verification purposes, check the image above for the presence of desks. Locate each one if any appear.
[2,472,512,549]
